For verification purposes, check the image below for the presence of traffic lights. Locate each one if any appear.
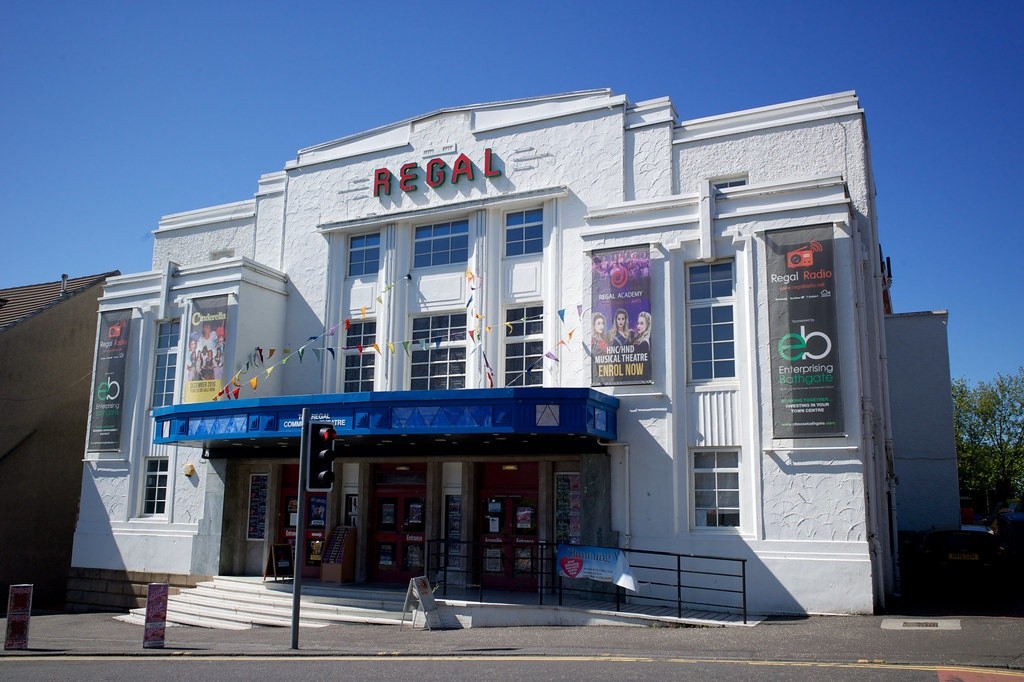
[306,421,337,492]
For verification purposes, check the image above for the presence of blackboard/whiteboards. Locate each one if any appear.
[248,475,267,539]
[264,543,294,578]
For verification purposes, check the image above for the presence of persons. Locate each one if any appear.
[187,324,225,381]
[591,309,651,356]
